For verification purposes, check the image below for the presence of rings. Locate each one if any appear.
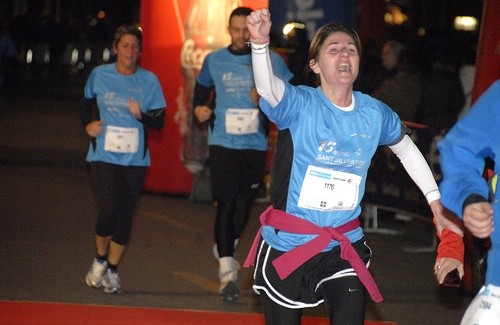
[437,264,442,270]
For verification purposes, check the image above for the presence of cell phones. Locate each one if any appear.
[444,268,461,287]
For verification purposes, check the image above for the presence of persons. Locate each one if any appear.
[432,78,500,325]
[81,25,168,294]
[266,23,476,205]
[192,4,302,305]
[432,154,495,285]
[243,7,467,325]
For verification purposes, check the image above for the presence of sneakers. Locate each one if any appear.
[218,277,241,303]
[84,257,123,295]
